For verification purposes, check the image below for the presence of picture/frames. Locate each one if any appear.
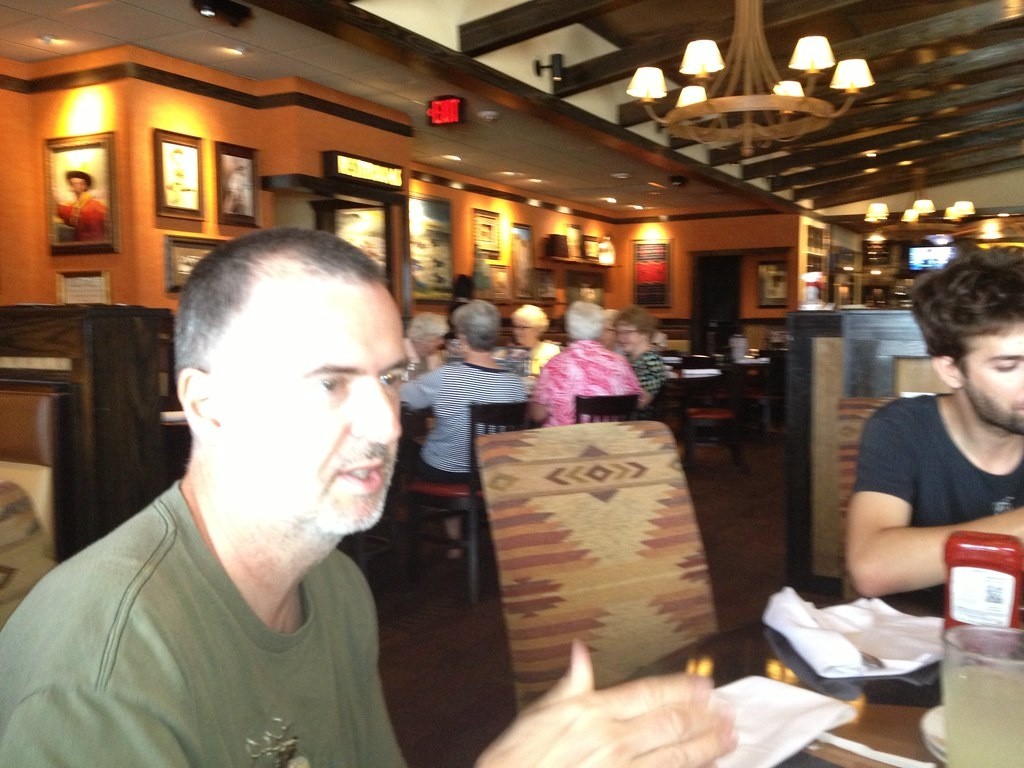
[42,130,121,257]
[471,206,502,261]
[408,190,457,305]
[163,233,228,295]
[534,267,557,301]
[582,234,603,262]
[490,263,513,305]
[54,269,112,304]
[628,237,676,309]
[564,268,606,307]
[511,222,537,301]
[213,140,264,229]
[567,223,583,259]
[153,127,206,223]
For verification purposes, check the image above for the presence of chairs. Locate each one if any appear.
[473,419,718,713]
[661,350,786,476]
[575,392,641,423]
[402,399,534,605]
[835,396,901,604]
[0,480,59,631]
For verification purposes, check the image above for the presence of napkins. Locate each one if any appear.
[761,586,945,678]
[713,674,857,768]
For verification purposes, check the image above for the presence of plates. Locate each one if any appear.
[660,357,682,363]
[920,705,947,763]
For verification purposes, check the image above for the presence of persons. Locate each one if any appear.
[844,253,1024,600]
[0,228,745,768]
[397,275,668,562]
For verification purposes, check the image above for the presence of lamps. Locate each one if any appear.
[534,53,565,82]
[864,165,976,224]
[625,0,875,159]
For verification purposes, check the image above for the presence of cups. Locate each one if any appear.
[941,626,1024,768]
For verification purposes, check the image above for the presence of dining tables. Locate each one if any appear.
[628,595,1024,768]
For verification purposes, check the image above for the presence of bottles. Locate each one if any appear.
[728,335,748,360]
[945,531,1023,632]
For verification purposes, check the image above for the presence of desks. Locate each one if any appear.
[662,368,725,409]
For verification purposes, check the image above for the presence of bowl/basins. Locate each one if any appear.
[713,354,724,361]
